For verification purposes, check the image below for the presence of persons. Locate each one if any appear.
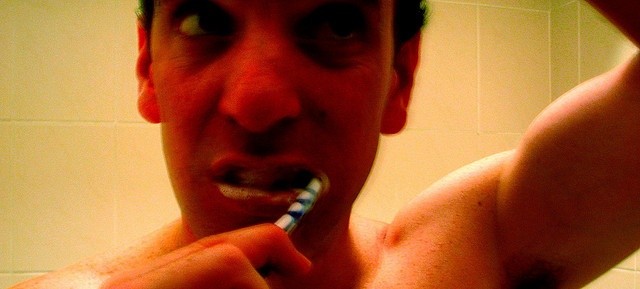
[1,0,640,288]
